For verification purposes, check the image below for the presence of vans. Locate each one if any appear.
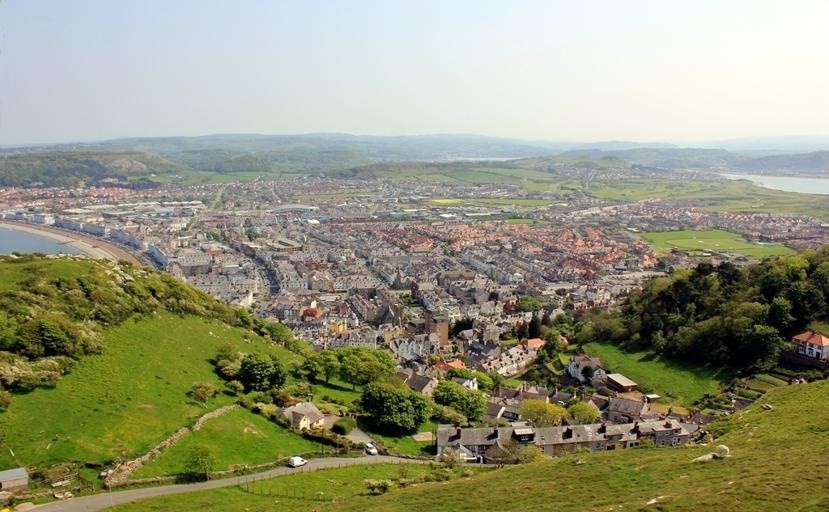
[289,457,307,468]
[365,444,377,455]
